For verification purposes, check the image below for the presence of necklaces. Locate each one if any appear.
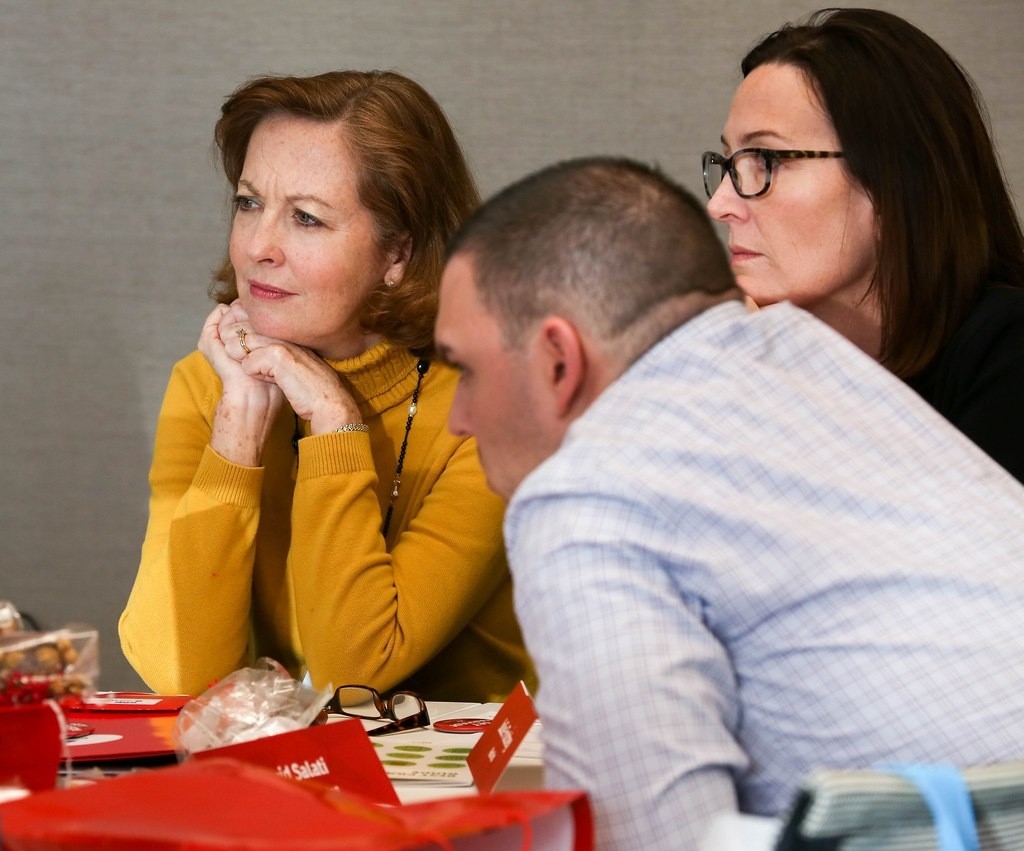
[292,358,431,538]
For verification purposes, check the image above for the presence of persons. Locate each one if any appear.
[435,156,1024,851]
[708,8,1024,484]
[117,68,541,702]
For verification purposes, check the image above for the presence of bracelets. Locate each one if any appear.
[334,424,370,432]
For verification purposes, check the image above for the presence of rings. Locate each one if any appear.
[236,328,251,353]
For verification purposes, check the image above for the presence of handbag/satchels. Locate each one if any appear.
[2,756,596,851]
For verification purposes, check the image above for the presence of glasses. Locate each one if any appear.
[702,147,844,200]
[308,684,431,735]
